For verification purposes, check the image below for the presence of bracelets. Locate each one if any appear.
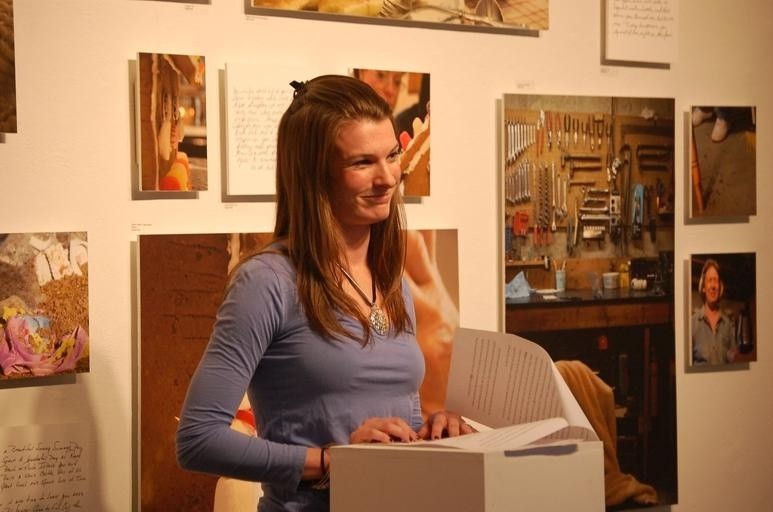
[317,441,333,481]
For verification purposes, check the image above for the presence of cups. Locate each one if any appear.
[603,272,619,290]
[554,270,566,292]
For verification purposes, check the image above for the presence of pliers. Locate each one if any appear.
[545,111,613,152]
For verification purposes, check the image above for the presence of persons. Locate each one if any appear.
[174,76,480,512]
[582,331,621,400]
[393,74,433,138]
[690,259,742,367]
[351,70,402,112]
[691,107,737,142]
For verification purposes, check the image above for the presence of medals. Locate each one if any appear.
[369,304,391,337]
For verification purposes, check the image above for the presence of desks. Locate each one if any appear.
[505,292,672,489]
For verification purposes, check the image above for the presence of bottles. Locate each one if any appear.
[734,308,753,354]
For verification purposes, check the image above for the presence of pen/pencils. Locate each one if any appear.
[553,260,567,271]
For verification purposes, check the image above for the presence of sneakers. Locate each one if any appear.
[710,117,734,142]
[690,106,714,127]
